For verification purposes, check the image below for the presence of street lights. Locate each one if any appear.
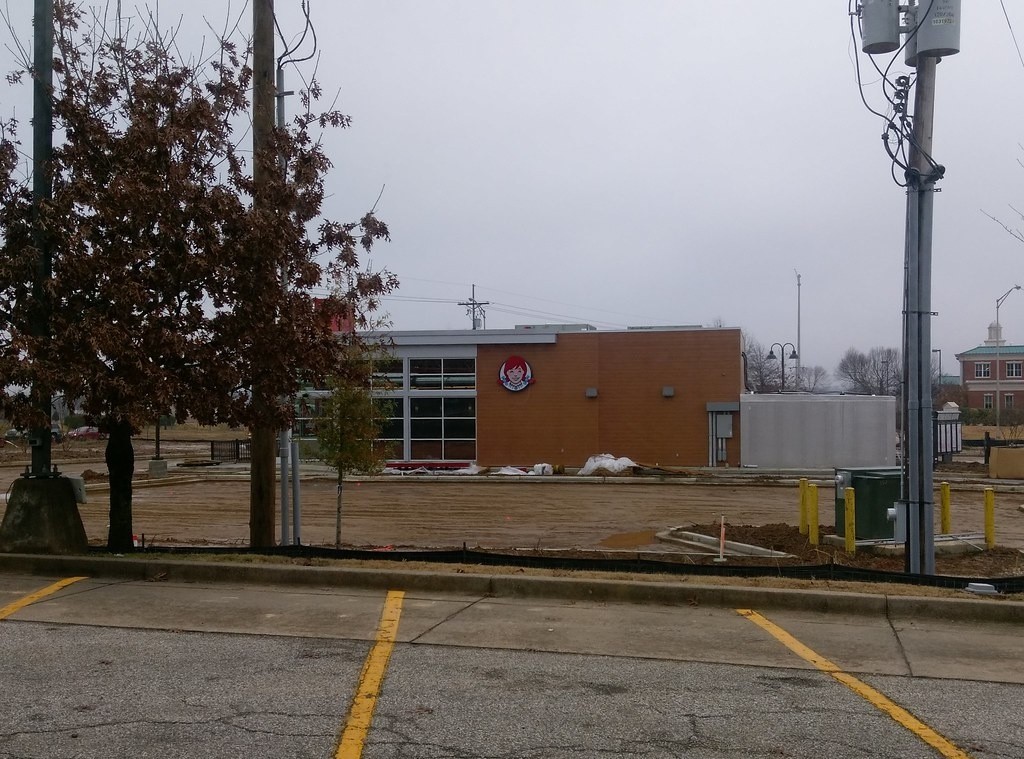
[764,343,801,390]
[995,285,1022,438]
[932,349,942,386]
[880,360,889,395]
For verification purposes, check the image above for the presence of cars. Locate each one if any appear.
[63,425,107,440]
[5,421,63,444]
